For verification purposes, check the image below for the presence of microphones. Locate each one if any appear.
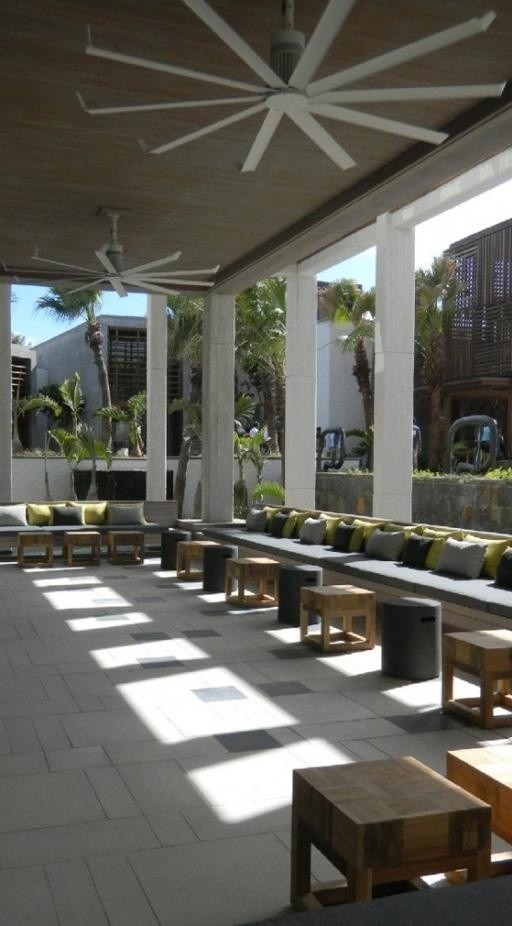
[176,539,221,582]
[299,585,376,652]
[64,531,101,566]
[107,530,145,565]
[442,628,512,729]
[225,556,280,608]
[17,532,53,567]
[445,746,512,883]
[289,752,492,908]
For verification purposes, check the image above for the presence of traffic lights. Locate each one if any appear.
[49,504,86,525]
[246,506,511,591]
[65,500,107,524]
[27,504,53,527]
[49,505,62,525]
[0,504,28,526]
[106,502,146,525]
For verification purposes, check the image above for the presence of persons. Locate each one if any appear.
[316,427,326,459]
[413,418,422,472]
[329,433,339,459]
[249,421,260,438]
[479,425,491,464]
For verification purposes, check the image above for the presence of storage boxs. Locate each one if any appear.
[204,501,511,651]
[0,500,179,562]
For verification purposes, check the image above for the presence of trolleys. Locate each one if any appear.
[76,2,508,175]
[30,209,222,299]
